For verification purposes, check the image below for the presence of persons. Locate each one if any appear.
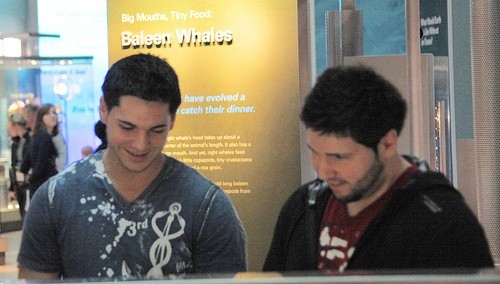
[263,66,495,276]
[8,102,68,220]
[16,53,247,280]
